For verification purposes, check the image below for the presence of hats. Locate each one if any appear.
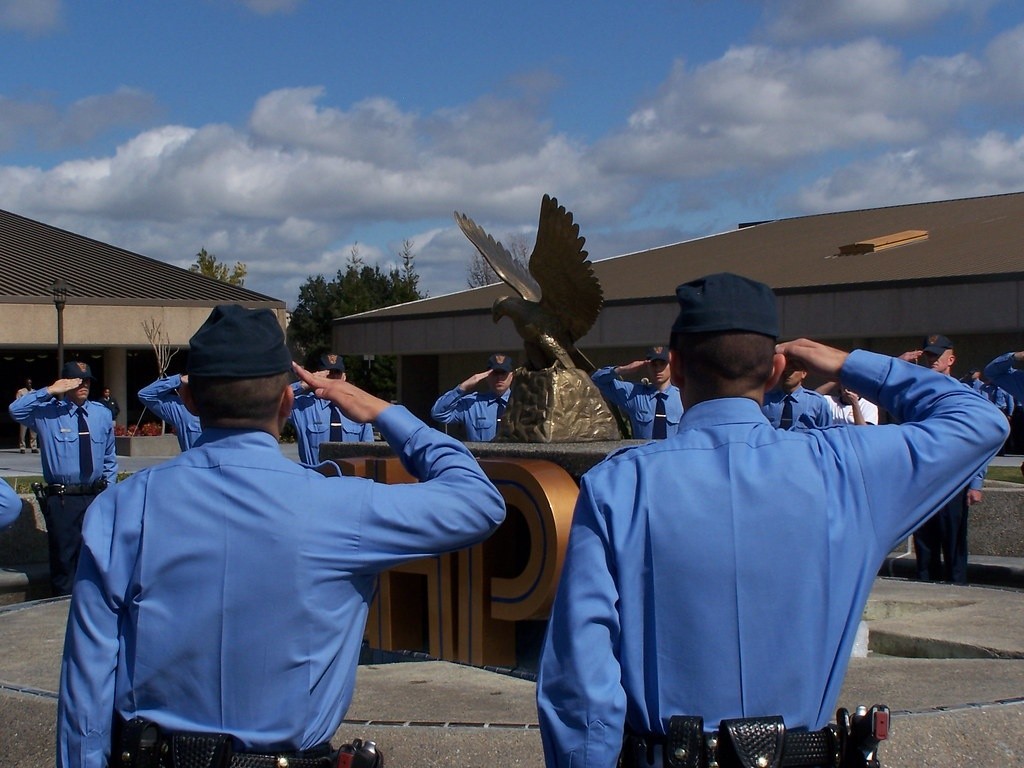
[672,273,779,337]
[969,368,980,374]
[923,334,954,355]
[488,354,512,372]
[186,304,291,377]
[313,354,344,371]
[61,361,94,380]
[646,346,669,362]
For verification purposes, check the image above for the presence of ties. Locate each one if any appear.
[496,399,504,433]
[329,403,342,441]
[76,407,94,477]
[779,396,793,430]
[651,394,667,439]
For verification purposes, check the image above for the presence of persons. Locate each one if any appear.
[136,371,202,452]
[431,354,515,442]
[16,378,40,454]
[55,305,507,768]
[534,271,1011,768]
[286,353,374,477]
[9,360,118,597]
[98,387,120,427]
[761,334,1024,587]
[0,477,22,528]
[590,347,685,439]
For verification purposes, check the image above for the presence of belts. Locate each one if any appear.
[231,752,333,768]
[45,484,99,495]
[707,733,831,768]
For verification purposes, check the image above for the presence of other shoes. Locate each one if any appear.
[20,449,25,453]
[32,448,39,453]
